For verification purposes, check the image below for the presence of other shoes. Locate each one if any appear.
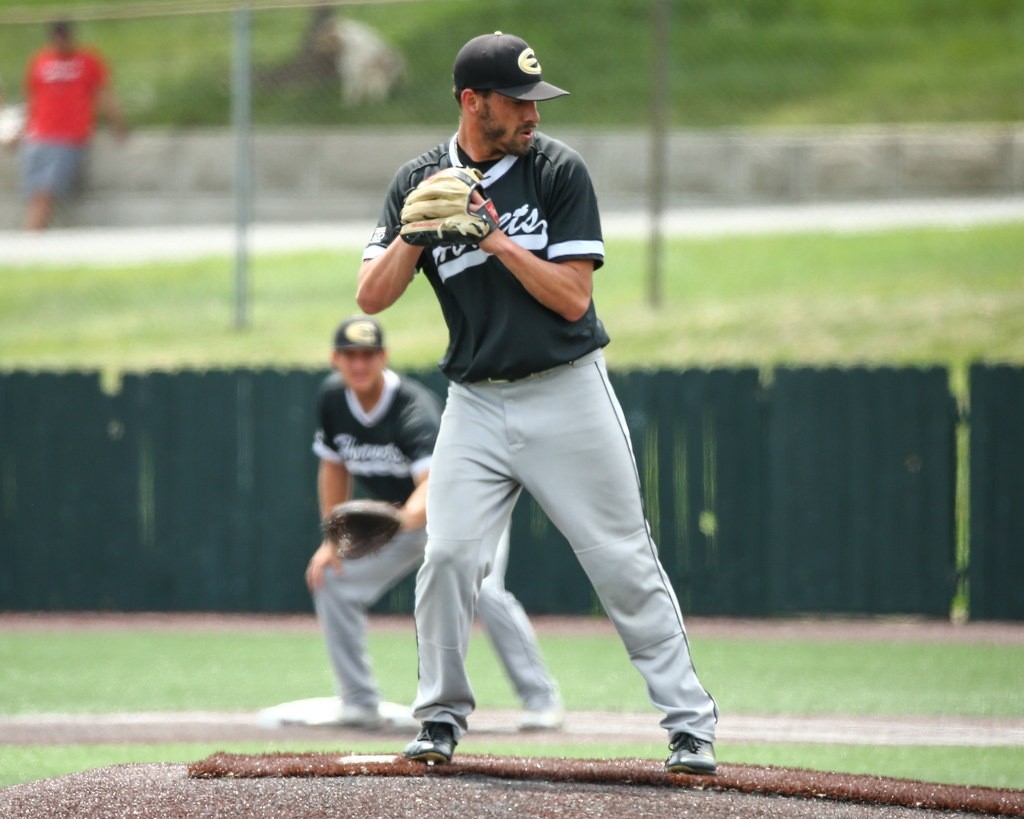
[304,701,384,731]
[516,707,569,733]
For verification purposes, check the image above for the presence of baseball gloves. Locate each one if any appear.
[320,499,402,563]
[398,164,499,246]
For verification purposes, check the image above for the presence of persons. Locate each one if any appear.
[19,21,130,231]
[298,8,408,112]
[306,322,561,737]
[356,31,720,772]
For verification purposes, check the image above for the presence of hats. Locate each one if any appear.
[450,31,572,102]
[332,317,386,351]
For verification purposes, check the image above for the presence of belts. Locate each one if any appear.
[487,347,602,385]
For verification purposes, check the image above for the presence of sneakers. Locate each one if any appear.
[664,733,718,776]
[401,721,457,766]
